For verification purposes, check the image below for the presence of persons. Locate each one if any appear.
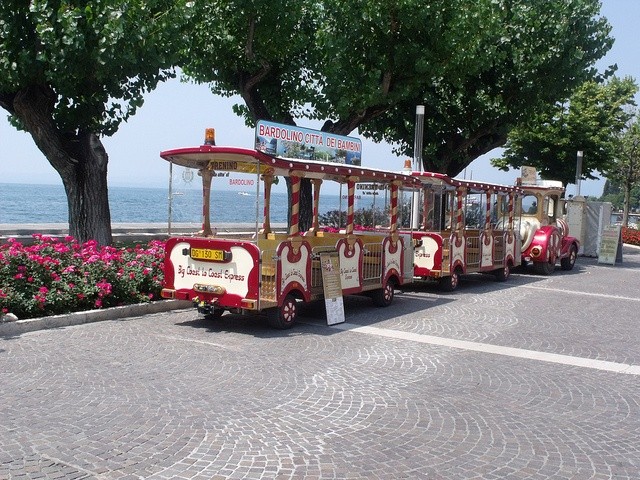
[527,200,537,214]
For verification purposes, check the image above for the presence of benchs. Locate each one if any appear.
[267,233,339,269]
[316,232,383,264]
[250,239,282,276]
[492,231,503,251]
[439,232,449,256]
[463,230,480,253]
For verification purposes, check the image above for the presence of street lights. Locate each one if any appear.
[574,150,584,203]
[410,105,425,233]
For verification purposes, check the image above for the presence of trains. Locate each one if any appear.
[160,128,580,329]
